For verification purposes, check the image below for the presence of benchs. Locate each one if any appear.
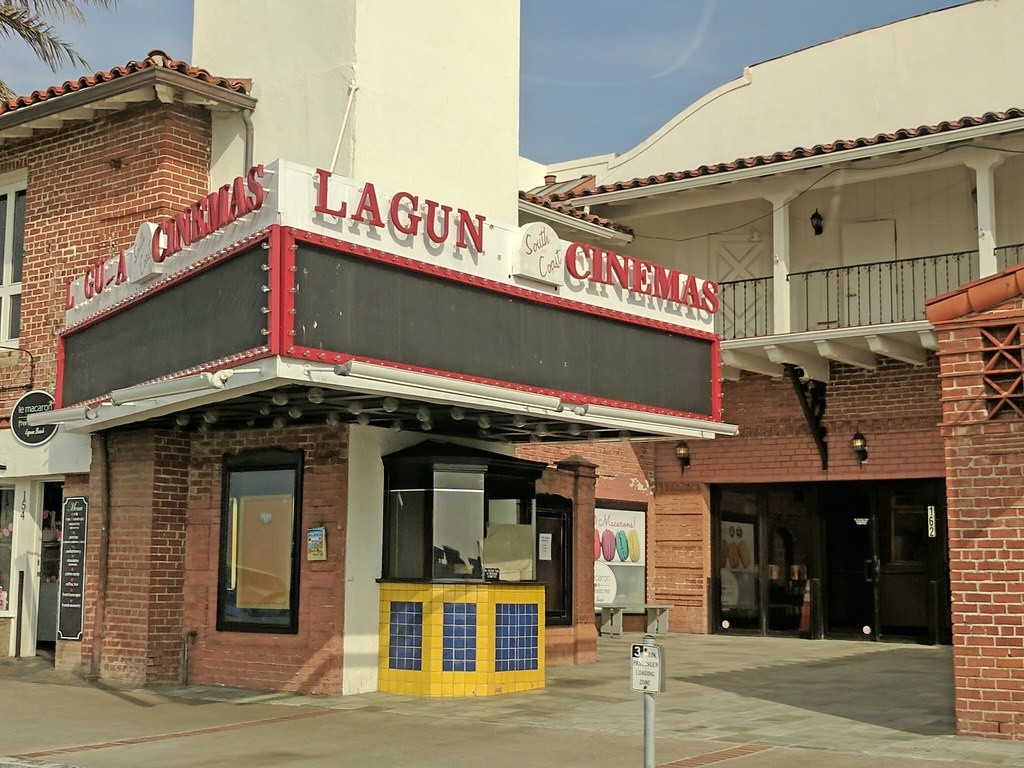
[594,604,674,639]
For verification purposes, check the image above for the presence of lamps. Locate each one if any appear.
[810,208,823,236]
[850,427,868,461]
[675,440,690,475]
